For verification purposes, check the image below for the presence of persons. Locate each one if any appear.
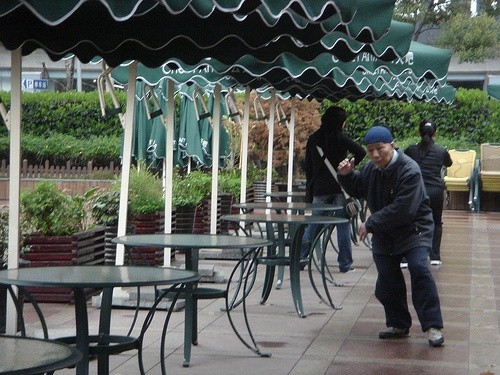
[298,105,368,273]
[399,119,453,268]
[337,126,444,347]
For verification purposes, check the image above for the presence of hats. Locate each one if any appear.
[365,126,394,145]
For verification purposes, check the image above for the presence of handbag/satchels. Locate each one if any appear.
[343,197,360,216]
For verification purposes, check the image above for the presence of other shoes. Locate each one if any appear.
[344,266,355,273]
[430,259,442,265]
[300,264,309,270]
[400,259,408,268]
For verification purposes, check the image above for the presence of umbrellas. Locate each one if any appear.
[0,0,457,335]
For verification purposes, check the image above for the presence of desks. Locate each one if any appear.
[113,233,275,366]
[220,215,349,319]
[0,264,201,375]
[231,201,345,290]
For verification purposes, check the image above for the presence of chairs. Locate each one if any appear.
[441,142,500,212]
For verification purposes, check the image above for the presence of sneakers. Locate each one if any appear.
[379,325,409,339]
[428,327,445,346]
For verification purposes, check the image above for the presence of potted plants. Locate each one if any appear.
[89,192,134,266]
[18,182,107,304]
[126,161,279,265]
